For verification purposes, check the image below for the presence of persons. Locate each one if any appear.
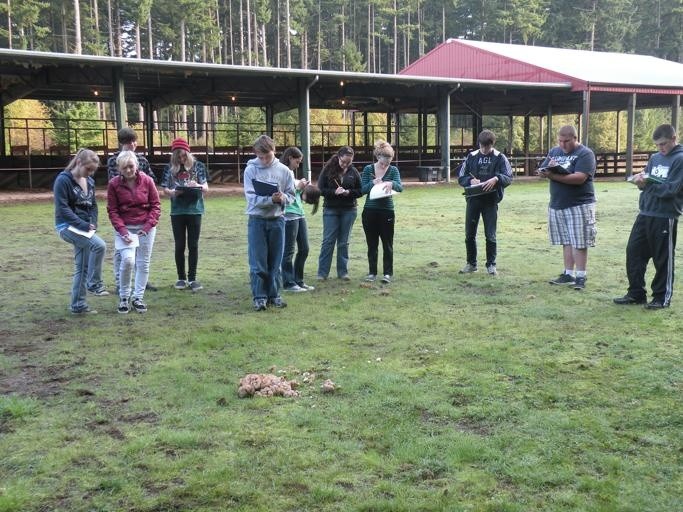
[458,131,513,277]
[161,138,209,292]
[53,147,110,315]
[107,128,158,292]
[613,123,682,310]
[361,140,403,284]
[106,150,161,314]
[244,135,296,311]
[316,146,363,282]
[279,147,315,294]
[538,125,597,291]
[295,179,320,216]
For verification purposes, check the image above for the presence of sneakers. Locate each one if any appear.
[364,274,377,282]
[253,296,287,311]
[645,298,669,309]
[458,263,478,274]
[174,280,203,292]
[549,269,586,290]
[71,307,98,315]
[380,274,393,283]
[486,264,497,275]
[282,283,316,292]
[146,283,157,291]
[87,288,110,296]
[613,295,647,304]
[316,276,329,281]
[340,273,351,281]
[117,298,147,314]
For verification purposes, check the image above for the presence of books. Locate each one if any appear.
[538,165,572,175]
[175,186,201,200]
[627,173,665,185]
[252,179,279,198]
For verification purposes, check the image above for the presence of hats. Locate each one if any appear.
[172,138,190,152]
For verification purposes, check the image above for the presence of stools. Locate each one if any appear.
[415,165,445,181]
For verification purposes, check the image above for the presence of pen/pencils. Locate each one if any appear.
[548,154,554,162]
[89,215,95,225]
[369,172,379,180]
[334,179,341,188]
[640,167,647,175]
[469,172,478,181]
